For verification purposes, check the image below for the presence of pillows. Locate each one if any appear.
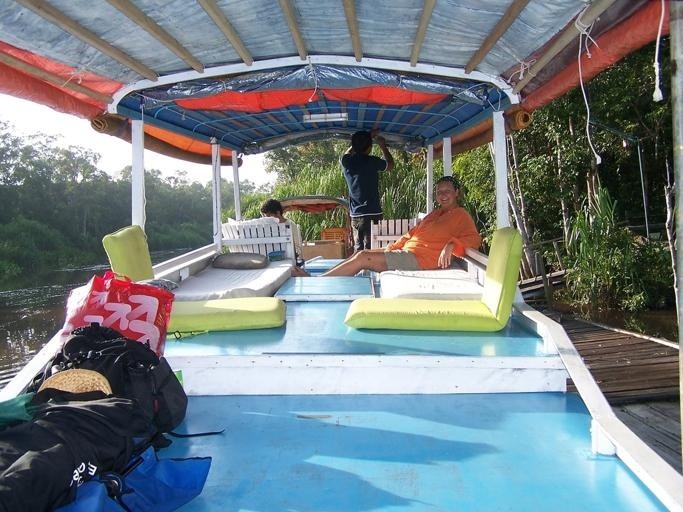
[214,253,266,271]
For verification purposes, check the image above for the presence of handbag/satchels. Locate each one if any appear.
[61,271,176,360]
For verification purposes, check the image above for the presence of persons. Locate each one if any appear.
[292,176,482,277]
[339,128,395,254]
[261,199,301,257]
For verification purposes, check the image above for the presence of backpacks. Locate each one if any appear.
[1,323,213,512]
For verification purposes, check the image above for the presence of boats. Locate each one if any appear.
[272,194,353,260]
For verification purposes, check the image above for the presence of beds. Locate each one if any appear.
[169,250,295,303]
[376,245,485,300]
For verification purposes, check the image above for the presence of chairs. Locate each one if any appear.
[343,225,524,332]
[102,223,287,331]
